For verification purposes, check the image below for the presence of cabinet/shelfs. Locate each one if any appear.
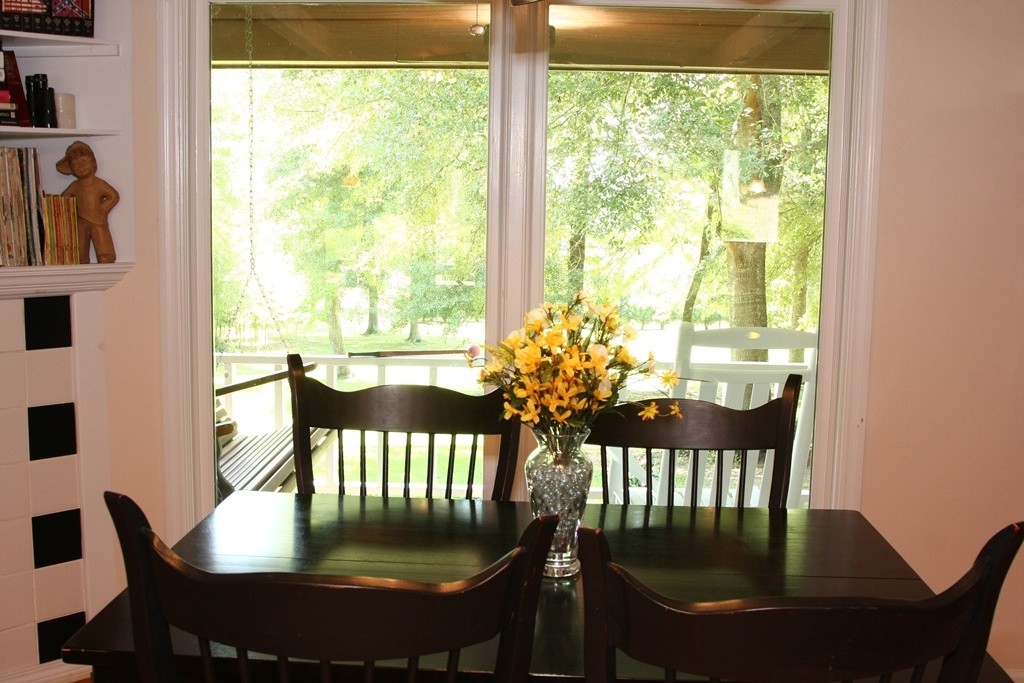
[0,29,137,298]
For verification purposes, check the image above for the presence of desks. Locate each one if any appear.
[61,491,1014,682]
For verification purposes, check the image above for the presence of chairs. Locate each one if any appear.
[102,489,561,683]
[578,520,1023,683]
[603,323,820,508]
[286,354,520,502]
[585,373,802,509]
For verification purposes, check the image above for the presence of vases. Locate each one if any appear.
[523,427,594,577]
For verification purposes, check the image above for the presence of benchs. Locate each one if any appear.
[215,362,333,493]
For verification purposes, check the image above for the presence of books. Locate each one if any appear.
[0,146,79,267]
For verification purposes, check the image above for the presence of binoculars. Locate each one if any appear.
[25,74,56,128]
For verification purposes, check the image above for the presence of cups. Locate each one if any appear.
[54,93,76,128]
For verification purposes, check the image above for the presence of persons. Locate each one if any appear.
[56,140,119,263]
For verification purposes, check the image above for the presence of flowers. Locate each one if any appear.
[463,291,682,466]
